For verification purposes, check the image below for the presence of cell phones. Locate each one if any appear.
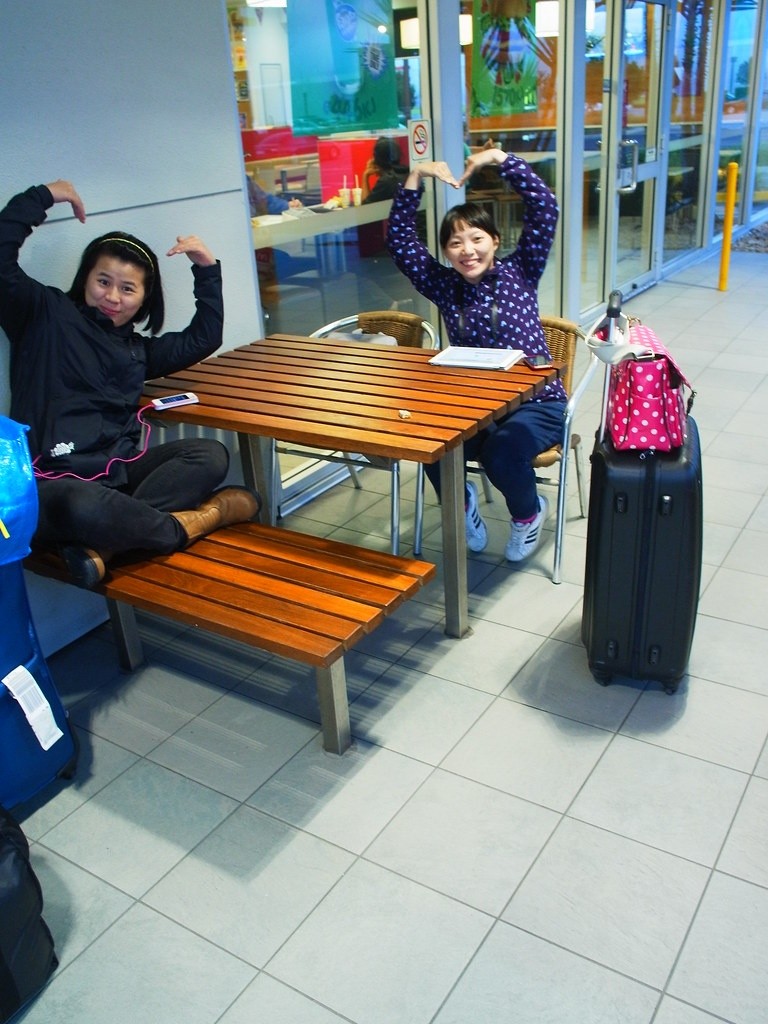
[152,392,199,410]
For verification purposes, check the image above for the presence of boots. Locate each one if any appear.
[170,485,262,550]
[61,540,116,585]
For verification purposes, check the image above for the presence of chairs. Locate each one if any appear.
[253,159,321,194]
[268,311,599,583]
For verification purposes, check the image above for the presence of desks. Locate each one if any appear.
[668,166,694,176]
[250,201,349,277]
[135,333,569,559]
[466,152,556,249]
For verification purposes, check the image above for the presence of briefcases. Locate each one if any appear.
[582,290,704,694]
[0,413,79,810]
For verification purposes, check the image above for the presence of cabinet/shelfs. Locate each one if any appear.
[234,69,252,130]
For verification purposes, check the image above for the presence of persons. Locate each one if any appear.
[483,133,502,188]
[1,180,263,588]
[248,175,302,336]
[463,121,472,191]
[357,136,426,313]
[388,149,568,560]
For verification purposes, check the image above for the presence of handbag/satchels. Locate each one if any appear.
[0,808,59,1024]
[586,310,696,451]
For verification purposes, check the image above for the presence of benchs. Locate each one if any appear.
[24,521,437,754]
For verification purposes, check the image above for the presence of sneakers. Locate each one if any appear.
[465,481,488,552]
[505,494,549,561]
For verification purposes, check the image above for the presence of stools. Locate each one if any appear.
[466,187,556,249]
[375,272,430,337]
[262,269,361,337]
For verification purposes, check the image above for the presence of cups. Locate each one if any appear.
[338,189,351,206]
[352,188,362,205]
